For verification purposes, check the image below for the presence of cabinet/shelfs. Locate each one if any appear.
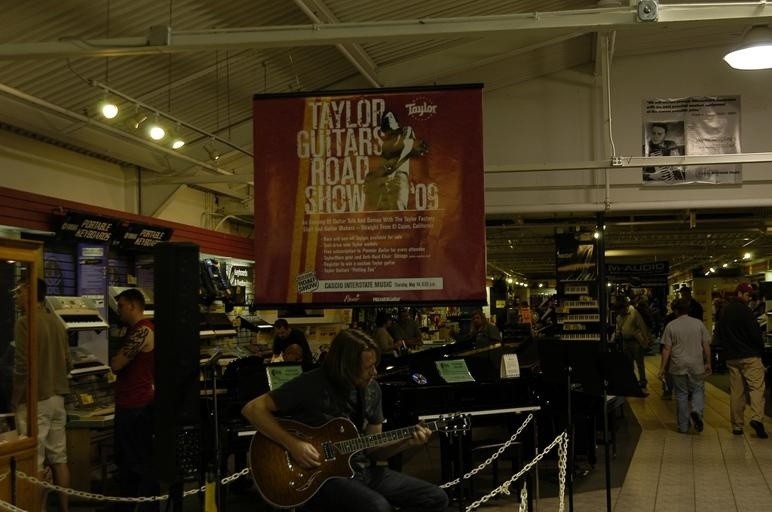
[0,235,44,512]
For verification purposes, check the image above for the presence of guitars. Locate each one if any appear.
[246,411,472,509]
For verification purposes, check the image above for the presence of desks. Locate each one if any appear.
[390,335,454,355]
[383,359,538,413]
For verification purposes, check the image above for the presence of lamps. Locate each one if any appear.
[146,114,165,141]
[166,127,187,149]
[204,143,222,161]
[722,25,772,70]
[98,100,118,119]
[125,113,146,130]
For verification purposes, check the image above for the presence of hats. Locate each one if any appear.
[737,282,760,294]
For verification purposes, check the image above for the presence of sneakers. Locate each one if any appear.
[691,410,704,433]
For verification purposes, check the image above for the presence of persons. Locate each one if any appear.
[375,110,416,211]
[718,284,769,440]
[643,122,686,183]
[471,310,502,350]
[658,299,712,434]
[680,288,703,321]
[271,320,313,372]
[375,310,403,361]
[11,276,74,512]
[392,309,423,346]
[660,299,693,401]
[613,300,651,388]
[110,288,158,512]
[269,344,303,368]
[241,329,449,512]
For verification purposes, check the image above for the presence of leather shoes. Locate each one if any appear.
[749,419,768,438]
[733,428,743,435]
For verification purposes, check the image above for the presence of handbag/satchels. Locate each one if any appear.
[711,304,730,374]
